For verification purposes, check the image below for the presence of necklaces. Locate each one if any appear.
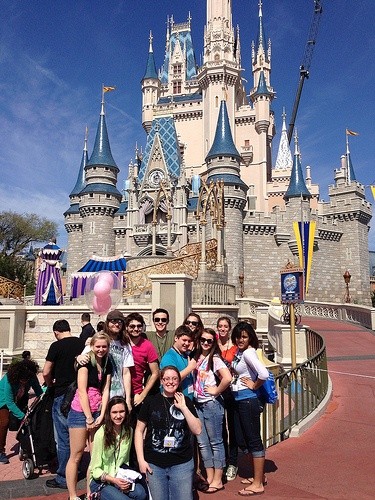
[172,344,184,360]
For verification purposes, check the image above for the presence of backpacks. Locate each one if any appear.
[210,350,235,410]
[59,381,77,418]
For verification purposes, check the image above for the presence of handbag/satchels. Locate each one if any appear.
[9,394,30,432]
[255,370,278,406]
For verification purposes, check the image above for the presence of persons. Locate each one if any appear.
[188,329,234,494]
[215,319,241,478]
[0,359,43,464]
[134,365,202,500]
[90,395,147,500]
[228,321,270,496]
[80,309,204,406]
[42,319,88,490]
[66,331,115,500]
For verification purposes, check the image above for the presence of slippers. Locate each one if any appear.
[198,479,225,493]
[241,475,267,485]
[238,488,265,495]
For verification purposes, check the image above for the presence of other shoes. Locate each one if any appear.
[241,449,248,455]
[0,452,10,464]
[226,465,238,481]
[46,479,68,488]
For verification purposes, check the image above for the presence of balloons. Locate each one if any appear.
[84,270,123,317]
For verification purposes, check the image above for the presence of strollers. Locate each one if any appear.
[15,379,53,479]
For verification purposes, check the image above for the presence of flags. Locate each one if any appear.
[346,129,359,136]
[103,87,115,92]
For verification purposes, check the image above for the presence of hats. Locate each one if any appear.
[107,310,125,320]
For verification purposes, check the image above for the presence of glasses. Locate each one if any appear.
[199,337,214,344]
[160,376,180,381]
[154,317,167,323]
[185,321,198,326]
[130,324,142,329]
